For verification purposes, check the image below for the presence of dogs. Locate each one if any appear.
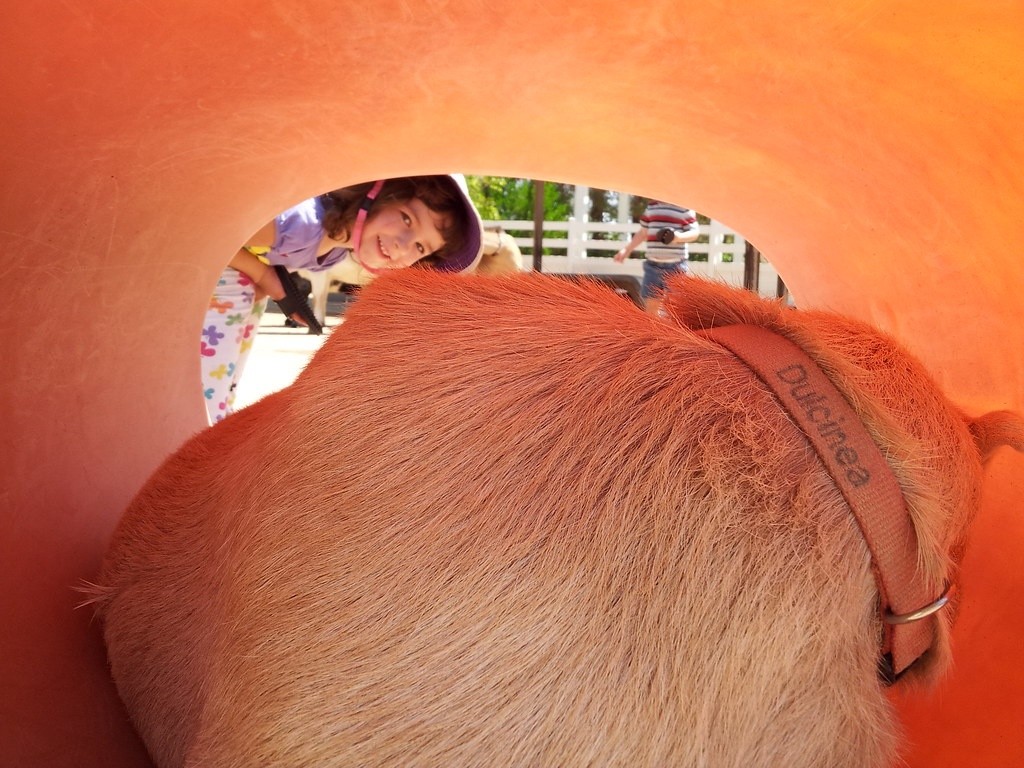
[72,259,1024,768]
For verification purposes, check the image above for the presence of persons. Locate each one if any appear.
[202,174,487,427]
[613,199,702,297]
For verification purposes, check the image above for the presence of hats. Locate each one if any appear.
[418,173,484,277]
